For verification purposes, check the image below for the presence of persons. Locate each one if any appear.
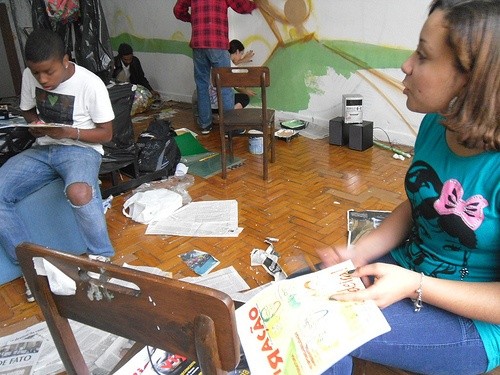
[173,0,259,139]
[192,40,257,125]
[0,28,116,303]
[285,0,500,375]
[106,43,161,100]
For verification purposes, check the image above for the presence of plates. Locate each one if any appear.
[280,120,306,130]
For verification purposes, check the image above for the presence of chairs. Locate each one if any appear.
[211,66,275,180]
[14,242,245,375]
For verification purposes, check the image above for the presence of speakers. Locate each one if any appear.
[328,93,373,151]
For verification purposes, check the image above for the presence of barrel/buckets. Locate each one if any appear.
[247,129,264,154]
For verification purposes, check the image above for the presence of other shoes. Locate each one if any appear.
[201,123,212,134]
[80,255,112,283]
[225,129,246,138]
[22,269,37,302]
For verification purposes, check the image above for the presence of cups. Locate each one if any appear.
[175,163,187,177]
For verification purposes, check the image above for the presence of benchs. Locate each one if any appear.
[0,177,105,286]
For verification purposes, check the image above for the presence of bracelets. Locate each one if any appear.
[410,271,424,313]
[77,127,80,141]
